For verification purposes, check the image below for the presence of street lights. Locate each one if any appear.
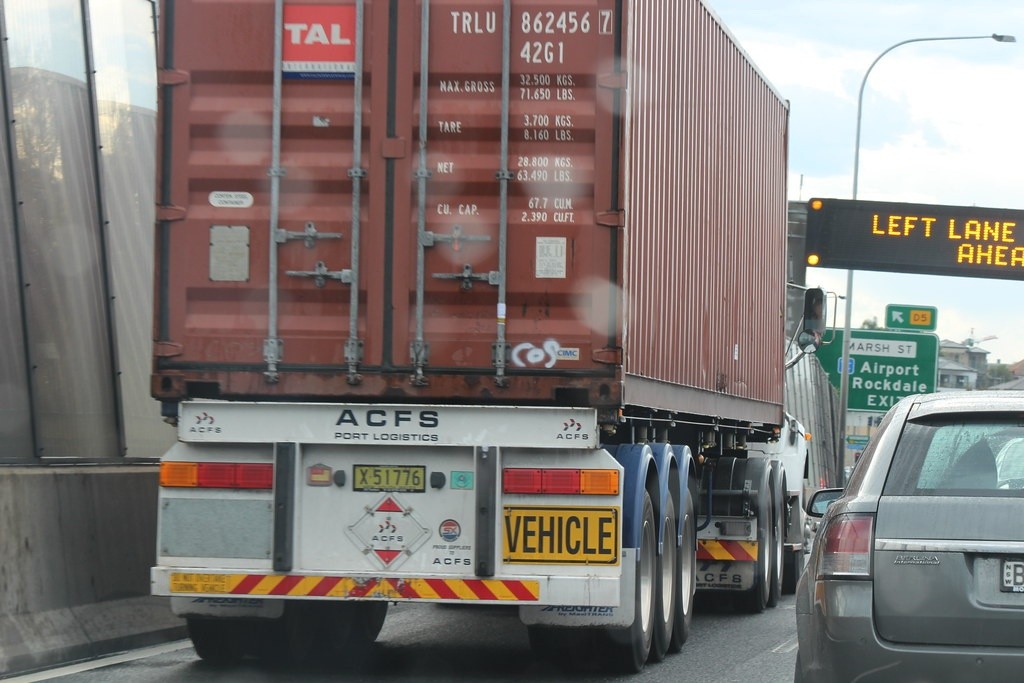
[838,34,1016,484]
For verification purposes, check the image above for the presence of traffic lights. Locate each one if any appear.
[876,416,882,427]
[867,416,872,426]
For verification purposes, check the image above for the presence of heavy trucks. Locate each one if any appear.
[151,0,836,674]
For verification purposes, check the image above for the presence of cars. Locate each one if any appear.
[794,390,1024,683]
